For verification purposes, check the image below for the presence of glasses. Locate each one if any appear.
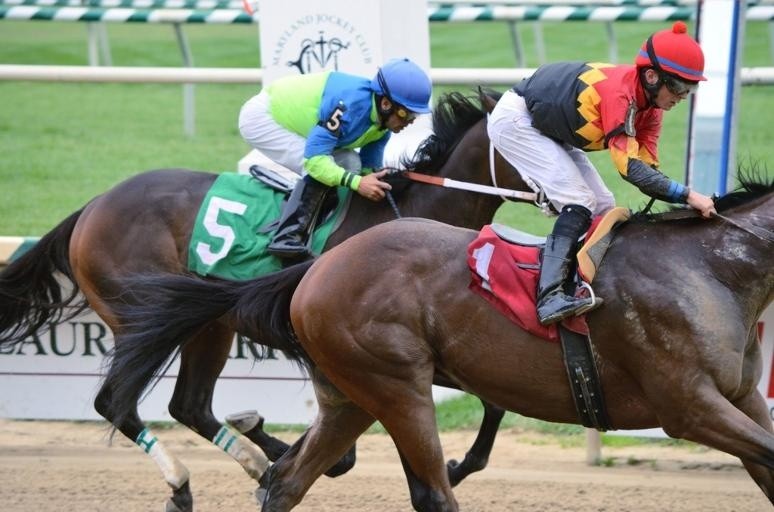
[647,34,699,95]
[377,68,416,122]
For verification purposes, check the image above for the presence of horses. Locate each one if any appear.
[0,83,558,512]
[97,155,774,512]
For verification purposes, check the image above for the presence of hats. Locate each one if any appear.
[370,57,432,113]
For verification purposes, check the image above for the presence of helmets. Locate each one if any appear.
[635,22,707,81]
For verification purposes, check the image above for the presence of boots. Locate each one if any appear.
[537,234,604,326]
[266,179,330,257]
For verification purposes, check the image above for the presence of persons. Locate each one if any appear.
[239,60,433,256]
[487,20,717,326]
